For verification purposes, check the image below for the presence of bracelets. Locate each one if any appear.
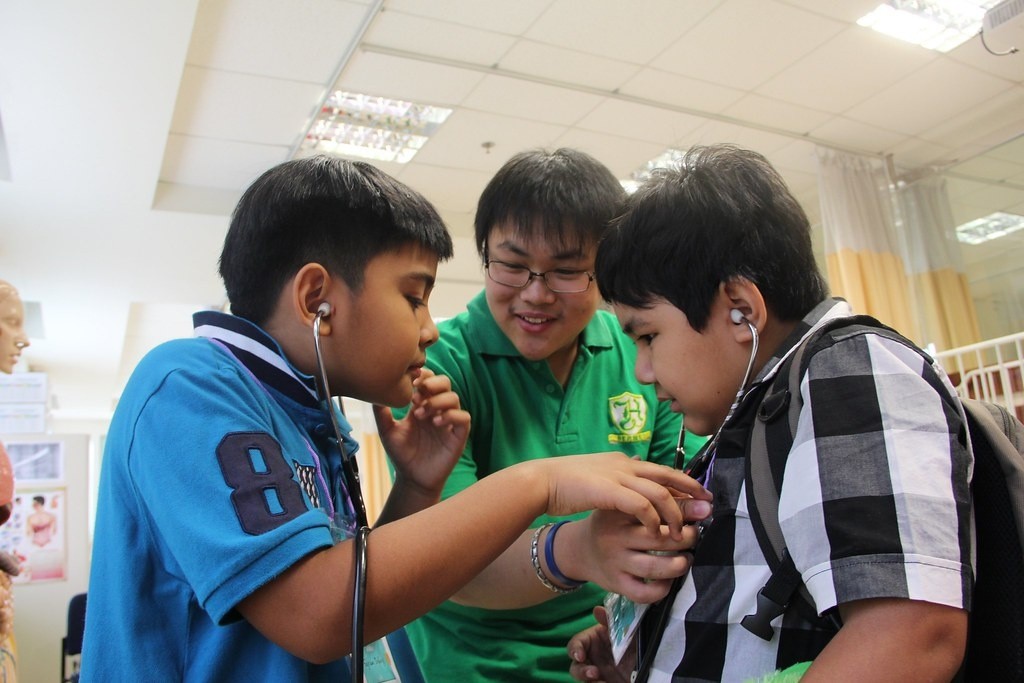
[530,522,584,595]
[544,520,588,587]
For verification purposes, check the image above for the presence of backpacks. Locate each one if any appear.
[742,315,1024,682]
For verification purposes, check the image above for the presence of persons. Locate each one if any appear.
[567,143,1024,683]
[0,277,31,683]
[77,153,713,683]
[385,147,708,683]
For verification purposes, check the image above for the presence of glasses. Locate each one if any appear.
[484,234,596,294]
